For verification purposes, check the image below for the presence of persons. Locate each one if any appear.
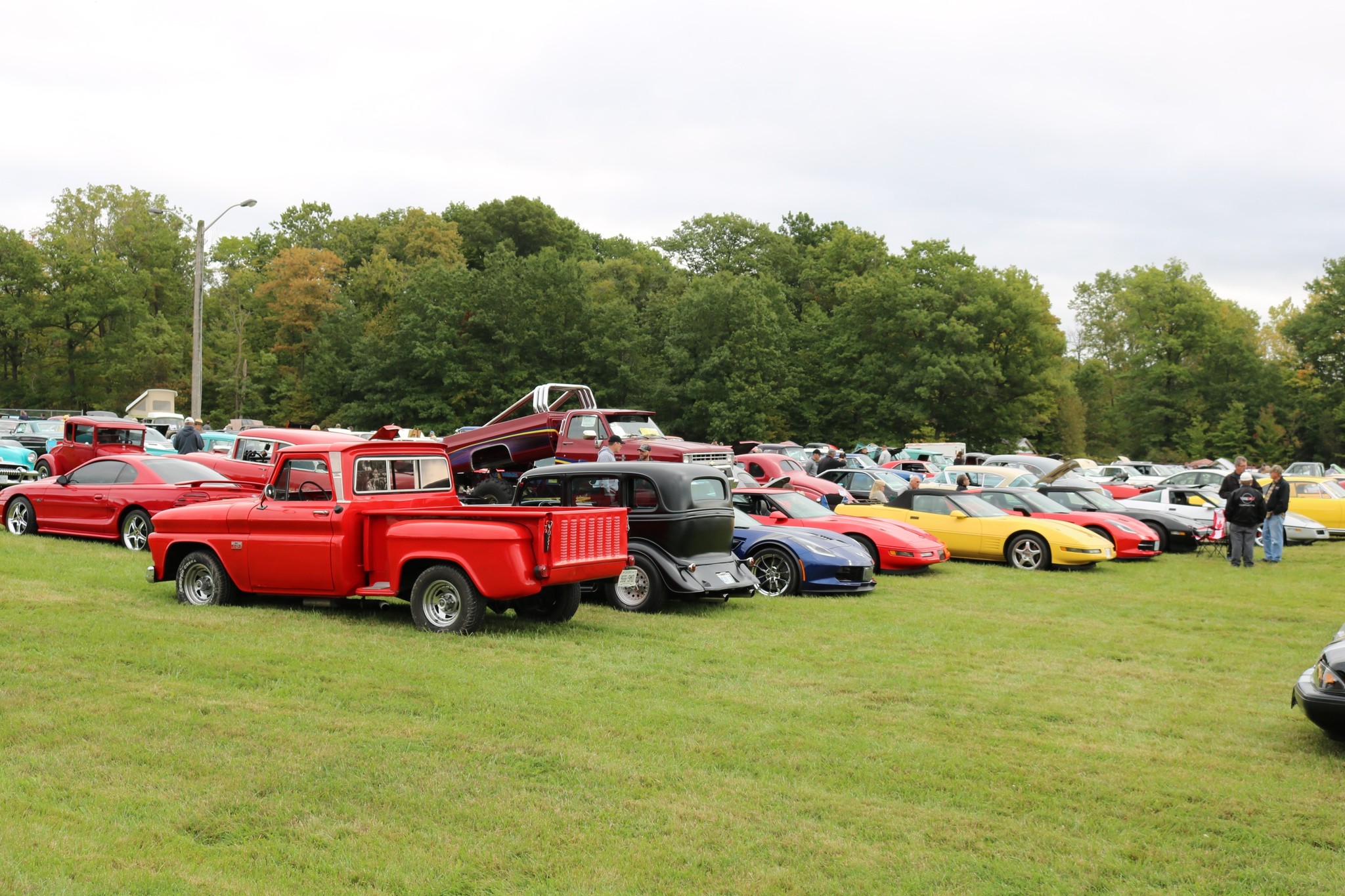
[1219,456,1263,562]
[634,444,654,490]
[898,475,920,497]
[1259,465,1290,564]
[593,435,626,504]
[954,451,965,465]
[1222,473,1268,567]
[711,440,723,446]
[19,409,436,455]
[753,445,891,486]
[1258,463,1269,473]
[1192,465,1198,469]
[1184,462,1189,466]
[956,474,970,490]
[869,479,889,503]
[1301,466,1309,475]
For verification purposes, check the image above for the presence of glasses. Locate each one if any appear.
[917,481,920,483]
[1238,465,1247,467]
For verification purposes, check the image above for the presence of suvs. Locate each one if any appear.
[441,383,735,507]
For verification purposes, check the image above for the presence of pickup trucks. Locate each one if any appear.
[145,441,634,636]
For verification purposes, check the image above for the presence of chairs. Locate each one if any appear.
[756,500,771,516]
[932,498,944,514]
[916,497,928,511]
[1192,508,1227,559]
[861,478,872,491]
[990,496,1001,508]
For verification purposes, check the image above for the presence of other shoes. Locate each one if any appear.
[1257,558,1277,564]
[1227,556,1232,562]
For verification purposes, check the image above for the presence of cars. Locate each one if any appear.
[513,462,761,612]
[0,410,424,551]
[1291,623,1345,742]
[721,440,1345,598]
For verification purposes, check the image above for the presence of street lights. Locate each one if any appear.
[147,200,257,426]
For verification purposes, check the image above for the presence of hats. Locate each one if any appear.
[1240,472,1253,481]
[882,445,887,448]
[813,449,821,454]
[637,444,651,452]
[347,426,353,429]
[430,430,435,435]
[1262,463,1266,466]
[61,414,69,422]
[141,418,147,422]
[184,417,194,423]
[828,449,836,454]
[608,435,626,444]
[194,419,204,425]
[336,424,341,427]
[1268,464,1282,474]
[713,439,717,441]
[862,447,867,449]
